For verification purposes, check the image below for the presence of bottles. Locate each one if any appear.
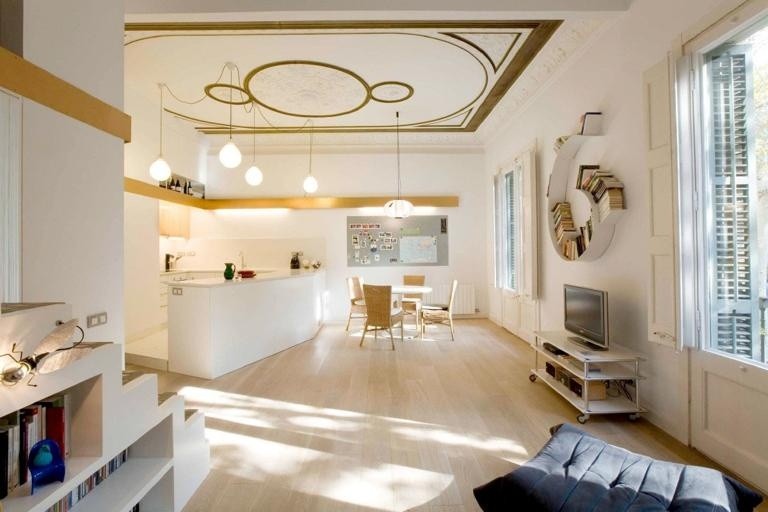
[171,178,175,191]
[176,178,181,192]
[184,178,188,194]
[188,182,193,196]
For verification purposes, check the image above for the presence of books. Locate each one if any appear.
[547,111,624,261]
[0,393,140,512]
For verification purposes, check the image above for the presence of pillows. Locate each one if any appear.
[473,424,763,512]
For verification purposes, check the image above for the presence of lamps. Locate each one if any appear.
[219,63,242,169]
[303,119,318,193]
[148,84,171,181]
[244,100,264,187]
[383,111,415,219]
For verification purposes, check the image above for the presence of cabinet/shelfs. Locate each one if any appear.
[0,343,175,512]
[530,330,651,424]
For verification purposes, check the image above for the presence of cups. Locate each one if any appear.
[302,259,310,270]
[312,258,322,271]
[158,180,166,188]
[234,274,242,282]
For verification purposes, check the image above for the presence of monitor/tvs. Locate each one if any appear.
[563,284,609,350]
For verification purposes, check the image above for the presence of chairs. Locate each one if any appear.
[346,275,457,350]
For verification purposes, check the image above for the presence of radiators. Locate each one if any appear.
[423,284,476,315]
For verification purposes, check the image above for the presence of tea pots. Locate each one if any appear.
[224,263,236,279]
